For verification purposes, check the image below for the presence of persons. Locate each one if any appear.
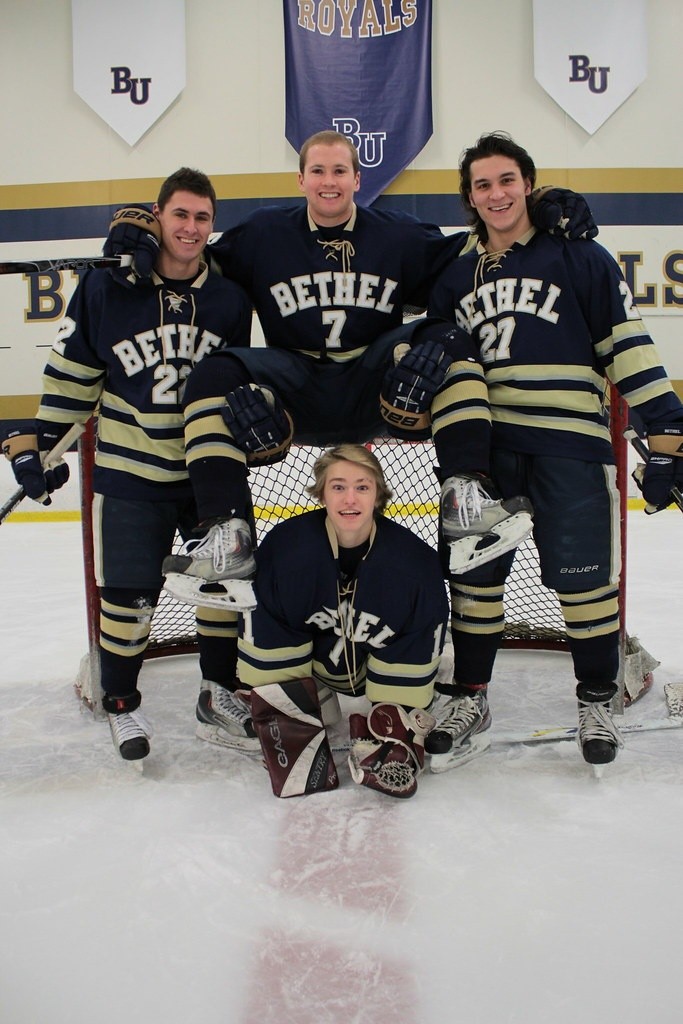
[237,443,451,800]
[429,134,683,765]
[0,166,258,760]
[103,130,599,581]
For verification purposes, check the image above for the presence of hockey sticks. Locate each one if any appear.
[486,681,683,744]
[622,425,683,514]
[0,253,134,276]
[0,422,87,527]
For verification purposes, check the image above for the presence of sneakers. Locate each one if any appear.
[162,517,258,612]
[108,711,151,772]
[423,679,492,773]
[434,467,533,575]
[577,697,624,779]
[196,679,263,756]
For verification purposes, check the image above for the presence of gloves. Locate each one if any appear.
[221,383,291,466]
[528,185,599,240]
[0,427,70,506]
[633,423,683,515]
[379,342,452,442]
[103,204,160,288]
